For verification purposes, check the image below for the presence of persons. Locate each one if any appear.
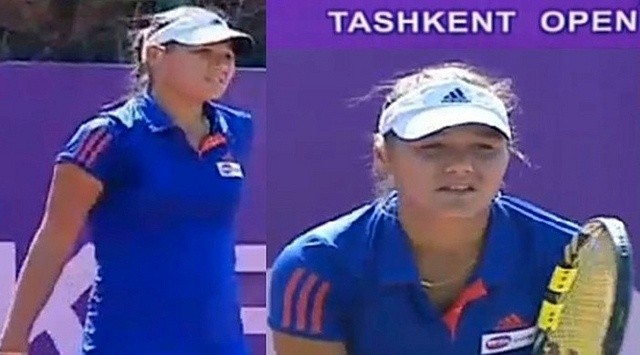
[1,4,256,354]
[266,67,584,355]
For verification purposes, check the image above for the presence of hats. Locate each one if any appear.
[145,11,255,56]
[378,79,512,142]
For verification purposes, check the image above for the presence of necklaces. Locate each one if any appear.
[419,261,477,288]
